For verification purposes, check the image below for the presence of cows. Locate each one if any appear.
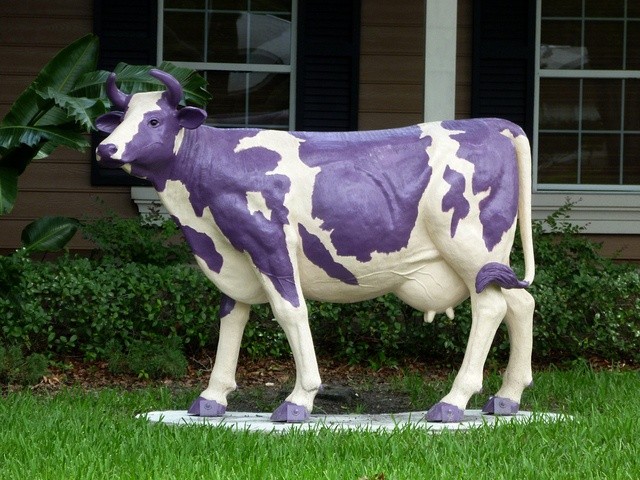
[94,68,536,423]
